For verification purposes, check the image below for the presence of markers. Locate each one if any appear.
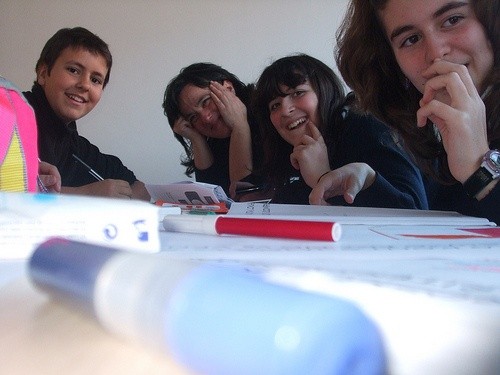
[26,237,388,375]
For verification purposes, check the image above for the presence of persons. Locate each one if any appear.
[310,0,500,228]
[22,26,151,202]
[162,62,272,198]
[38,160,61,192]
[229,53,346,205]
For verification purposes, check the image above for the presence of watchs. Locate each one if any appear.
[463,149,500,197]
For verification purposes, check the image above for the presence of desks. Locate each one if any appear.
[0,201,500,375]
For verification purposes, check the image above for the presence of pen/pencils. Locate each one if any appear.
[227,186,263,197]
[163,213,341,242]
[68,153,106,181]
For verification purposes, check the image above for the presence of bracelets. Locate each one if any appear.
[316,172,329,184]
